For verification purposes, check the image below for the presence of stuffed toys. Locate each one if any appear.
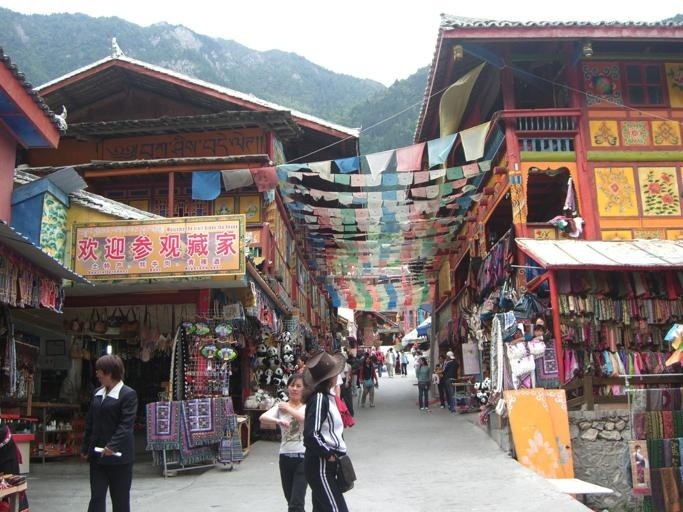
[242,340,296,410]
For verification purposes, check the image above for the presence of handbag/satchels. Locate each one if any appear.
[335,455,360,494]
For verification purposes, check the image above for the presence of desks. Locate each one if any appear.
[547,477,614,509]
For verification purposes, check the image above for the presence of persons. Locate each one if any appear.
[531,324,553,342]
[0,419,28,511]
[339,344,459,415]
[299,350,350,512]
[78,354,138,512]
[504,327,534,346]
[259,373,310,512]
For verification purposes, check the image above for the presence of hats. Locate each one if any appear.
[301,350,346,388]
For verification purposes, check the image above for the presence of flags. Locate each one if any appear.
[190,120,492,313]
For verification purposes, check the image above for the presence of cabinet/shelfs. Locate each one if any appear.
[27,404,83,467]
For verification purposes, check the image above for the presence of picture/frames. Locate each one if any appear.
[46,339,66,356]
